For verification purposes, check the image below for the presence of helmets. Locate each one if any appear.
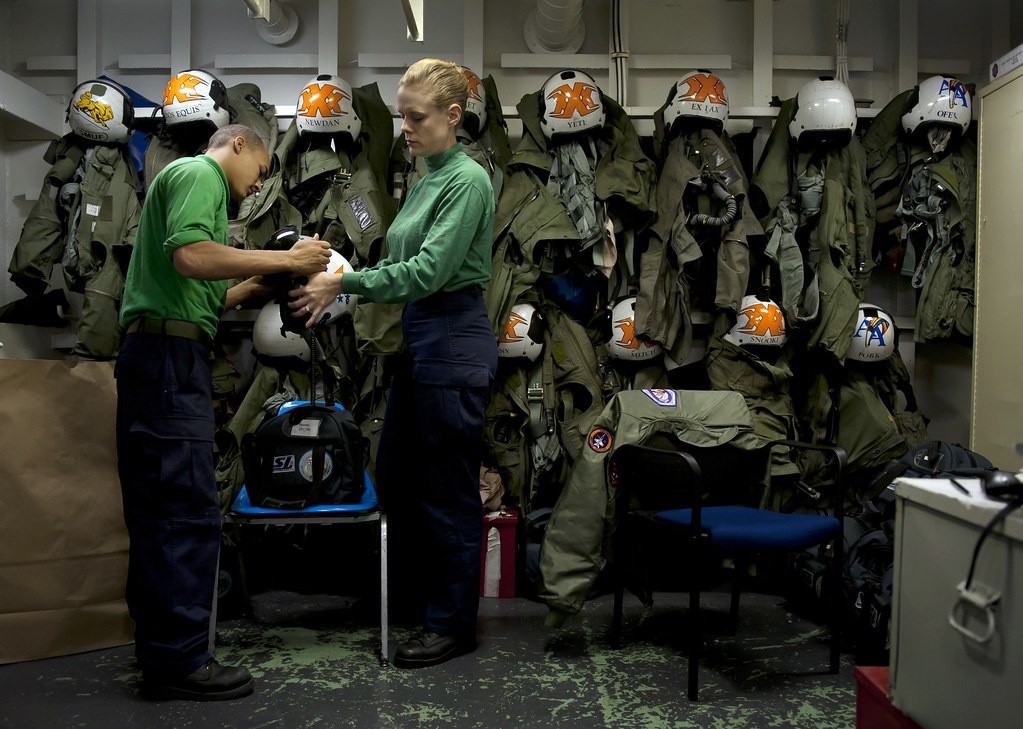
[900,75,972,139]
[163,69,231,131]
[723,295,786,354]
[601,297,663,361]
[66,79,136,143]
[664,70,729,137]
[495,303,545,362]
[789,75,857,150]
[540,70,605,139]
[460,65,487,133]
[845,303,895,361]
[253,300,310,370]
[286,234,358,324]
[296,74,362,144]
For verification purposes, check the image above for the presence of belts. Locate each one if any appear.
[127,317,207,341]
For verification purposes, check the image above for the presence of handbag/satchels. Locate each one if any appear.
[243,402,366,508]
[783,439,997,655]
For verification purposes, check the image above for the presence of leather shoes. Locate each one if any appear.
[394,626,477,668]
[152,651,254,697]
[137,631,219,671]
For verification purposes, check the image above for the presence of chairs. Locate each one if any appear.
[538,389,848,700]
[207,400,390,671]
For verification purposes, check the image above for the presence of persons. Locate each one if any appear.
[113,124,332,701]
[287,57,498,667]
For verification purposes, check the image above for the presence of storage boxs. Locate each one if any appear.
[886,478,1023,729]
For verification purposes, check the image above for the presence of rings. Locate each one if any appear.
[305,306,310,312]
